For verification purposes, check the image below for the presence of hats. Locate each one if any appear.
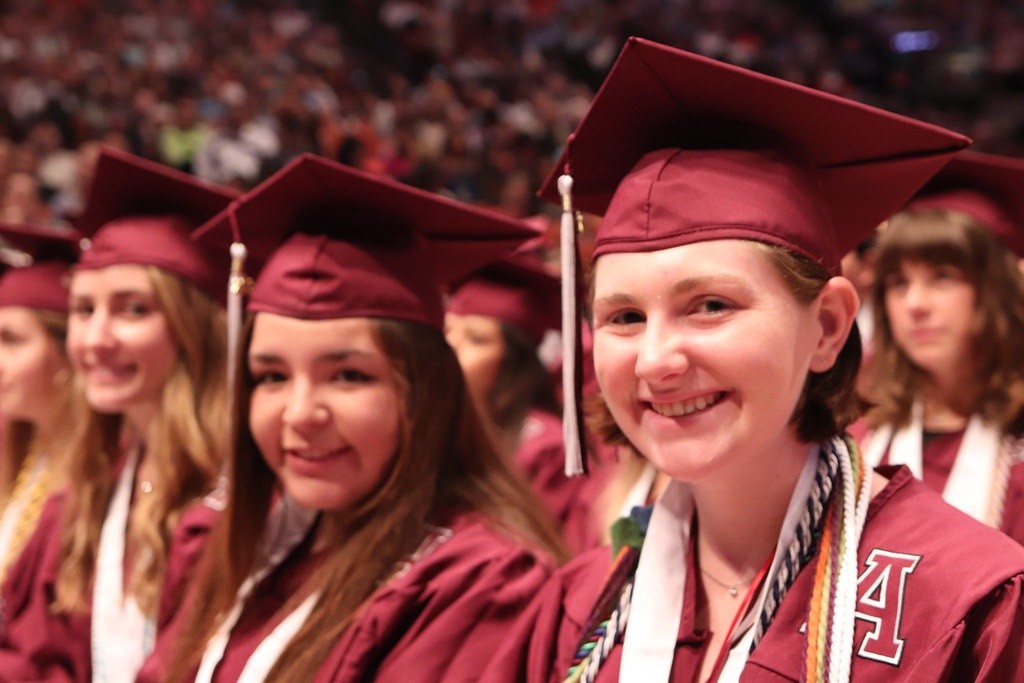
[192,154,547,404]
[73,145,242,309]
[453,255,564,340]
[538,38,974,478]
[880,156,1023,258]
[0,220,85,313]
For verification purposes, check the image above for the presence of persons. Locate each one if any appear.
[467,36,1023,683]
[0,0,1024,683]
